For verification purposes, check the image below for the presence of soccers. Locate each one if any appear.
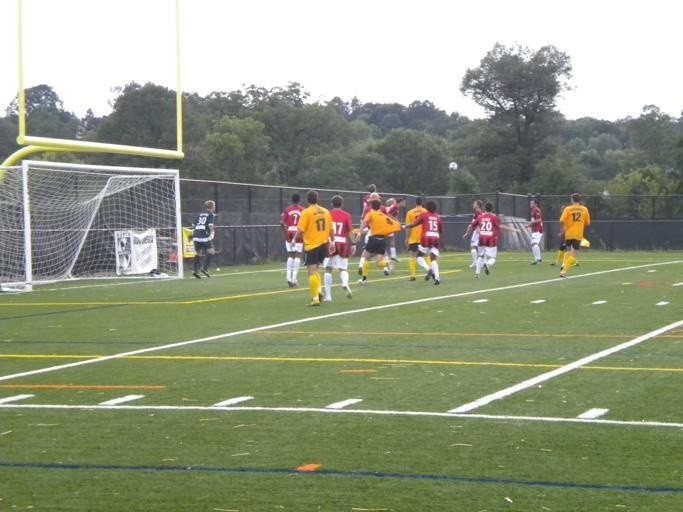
[449,162,457,172]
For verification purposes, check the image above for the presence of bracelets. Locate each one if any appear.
[351,243,356,245]
[291,238,294,246]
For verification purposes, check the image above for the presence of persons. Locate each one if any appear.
[280,194,304,288]
[356,182,443,285]
[462,203,522,279]
[551,204,590,267]
[468,199,488,268]
[324,196,356,301]
[525,199,543,266]
[558,193,590,278]
[190,200,219,278]
[289,188,335,306]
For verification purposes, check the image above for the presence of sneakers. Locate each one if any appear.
[340,286,353,300]
[191,269,210,279]
[469,261,493,279]
[553,260,582,277]
[532,256,544,264]
[304,294,332,306]
[286,279,299,288]
[358,257,440,286]
